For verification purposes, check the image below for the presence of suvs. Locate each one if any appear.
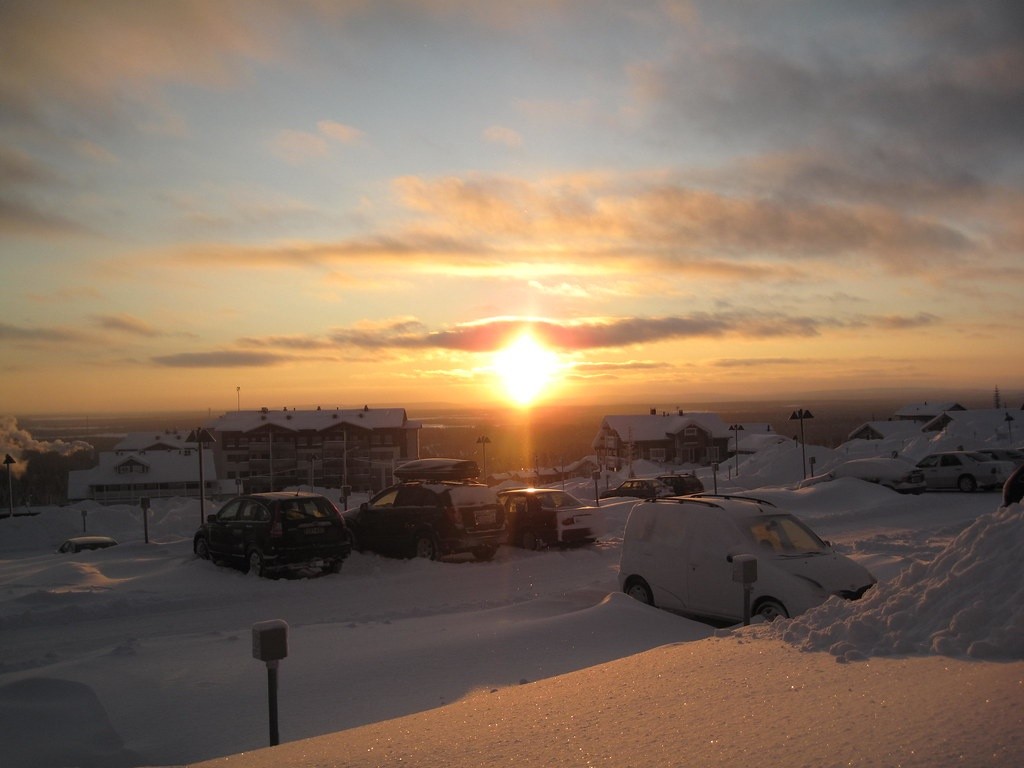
[654,473,705,495]
[194,492,354,579]
[340,457,510,562]
[599,478,676,499]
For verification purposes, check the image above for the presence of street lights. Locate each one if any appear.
[728,423,745,475]
[1004,412,1015,448]
[3,455,16,517]
[788,409,815,479]
[183,427,216,526]
[476,435,491,485]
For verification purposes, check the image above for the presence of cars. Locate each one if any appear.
[999,461,1024,509]
[976,448,1024,467]
[617,493,879,628]
[798,457,928,496]
[915,450,1016,494]
[55,535,119,554]
[496,486,609,551]
